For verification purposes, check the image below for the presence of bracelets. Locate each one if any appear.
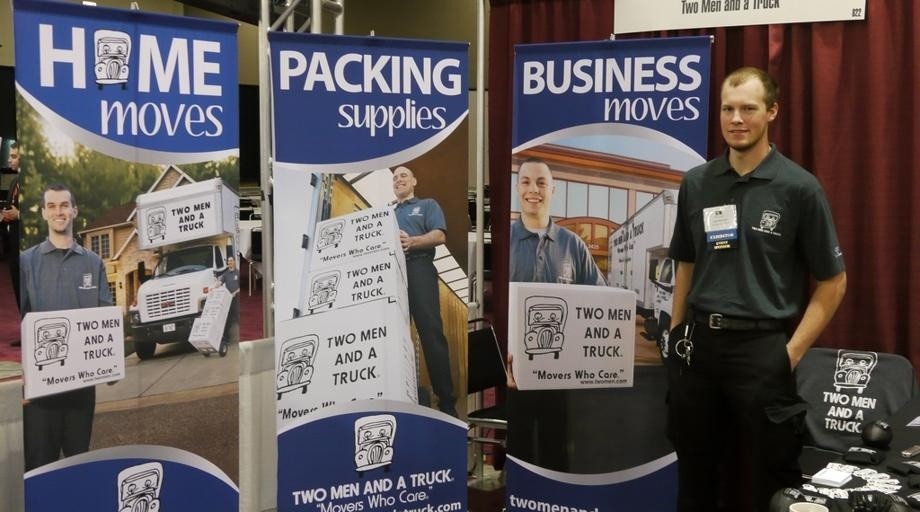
[17,210,20,221]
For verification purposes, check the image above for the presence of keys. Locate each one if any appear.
[685,346,692,366]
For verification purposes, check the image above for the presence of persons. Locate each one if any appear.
[389,165,459,419]
[217,256,241,342]
[668,61,849,511]
[0,142,25,347]
[21,184,116,471]
[511,161,609,471]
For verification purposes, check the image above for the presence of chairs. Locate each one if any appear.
[770,344,918,510]
[470,320,506,425]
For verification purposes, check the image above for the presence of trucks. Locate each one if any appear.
[606,190,679,361]
[128,178,242,358]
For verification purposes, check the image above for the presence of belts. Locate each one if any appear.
[404,249,433,262]
[686,310,771,332]
